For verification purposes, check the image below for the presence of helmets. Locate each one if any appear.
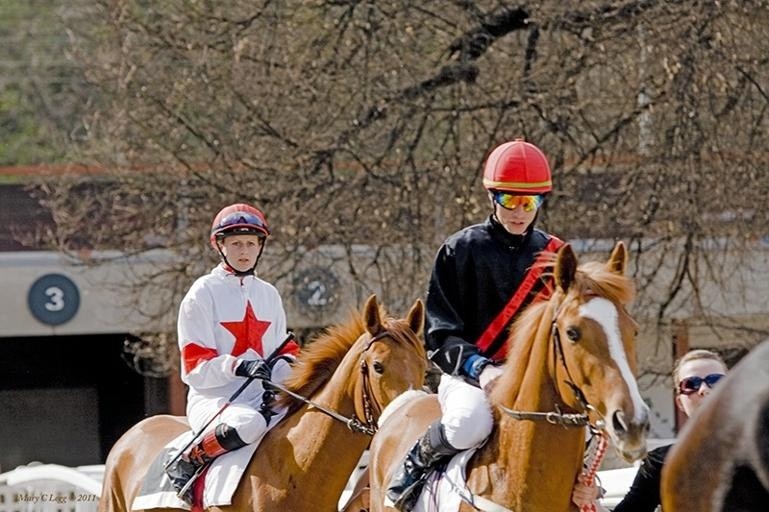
[481,137,553,192]
[209,203,270,245]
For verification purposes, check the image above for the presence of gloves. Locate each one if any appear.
[477,363,504,385]
[233,358,272,381]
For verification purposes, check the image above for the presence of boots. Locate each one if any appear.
[383,416,472,512]
[165,421,251,507]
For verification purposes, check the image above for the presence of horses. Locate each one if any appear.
[660,339,769,511]
[369,241,651,512]
[96,294,428,511]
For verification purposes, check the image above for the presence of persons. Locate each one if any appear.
[165,204,299,506]
[610,349,769,512]
[384,139,570,512]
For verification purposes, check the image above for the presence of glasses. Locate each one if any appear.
[219,212,263,225]
[675,373,725,395]
[495,191,549,212]
